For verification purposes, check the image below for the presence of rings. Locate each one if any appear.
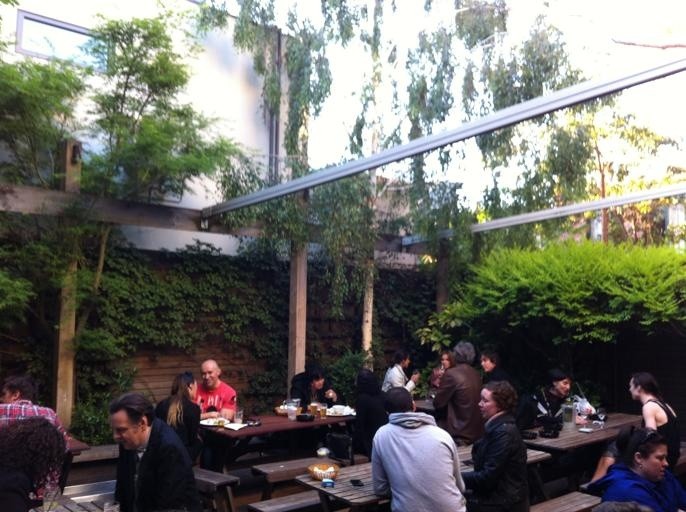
[328,393,332,397]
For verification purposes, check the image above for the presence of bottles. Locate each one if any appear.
[561,398,575,429]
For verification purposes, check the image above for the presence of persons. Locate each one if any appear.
[381,350,420,415]
[461,379,530,511]
[587,424,686,512]
[153,369,203,467]
[289,368,347,457]
[426,350,457,399]
[109,392,200,511]
[0,376,73,492]
[191,359,256,469]
[371,386,468,511]
[432,339,482,447]
[578,367,681,490]
[516,365,573,429]
[479,347,512,384]
[0,414,68,511]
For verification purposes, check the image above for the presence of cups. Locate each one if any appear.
[287,402,297,423]
[320,404,327,419]
[317,448,330,464]
[233,407,244,424]
[311,404,318,416]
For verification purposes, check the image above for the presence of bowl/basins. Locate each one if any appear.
[308,462,340,480]
[297,415,315,421]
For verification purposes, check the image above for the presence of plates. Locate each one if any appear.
[326,412,347,417]
[200,418,231,426]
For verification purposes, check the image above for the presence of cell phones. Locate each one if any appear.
[351,480,364,486]
